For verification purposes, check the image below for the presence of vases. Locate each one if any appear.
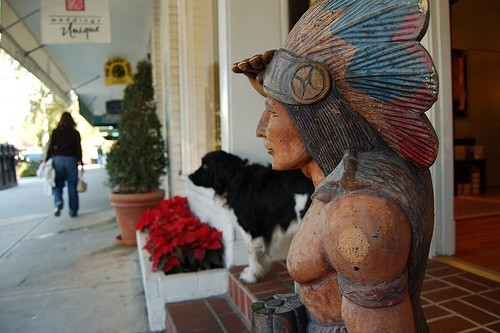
[136,230,228,333]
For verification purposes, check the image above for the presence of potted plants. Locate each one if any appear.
[106,62,169,246]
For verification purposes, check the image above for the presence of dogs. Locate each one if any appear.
[189,150,315,282]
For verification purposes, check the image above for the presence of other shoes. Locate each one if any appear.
[54,202,77,217]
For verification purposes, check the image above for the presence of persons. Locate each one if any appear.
[45,112,84,216]
[97,146,104,165]
[232,0,439,333]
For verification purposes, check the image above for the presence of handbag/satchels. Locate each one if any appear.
[76,164,87,192]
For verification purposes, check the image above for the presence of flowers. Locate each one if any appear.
[136,195,223,275]
